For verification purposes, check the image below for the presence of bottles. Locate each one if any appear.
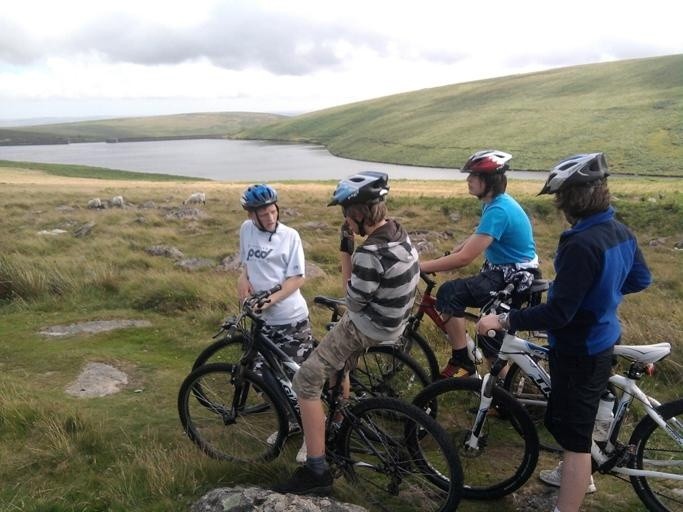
[464,330,482,362]
[594,388,617,441]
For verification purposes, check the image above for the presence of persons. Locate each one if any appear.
[418,148,541,419]
[472,152,652,511]
[270,171,422,495]
[235,183,314,464]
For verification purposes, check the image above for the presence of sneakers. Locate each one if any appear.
[265,421,301,445]
[270,465,334,497]
[439,357,479,378]
[538,460,598,495]
[295,435,307,463]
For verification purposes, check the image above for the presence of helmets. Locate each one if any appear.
[534,152,610,198]
[326,170,389,207]
[239,184,278,209]
[459,149,512,174]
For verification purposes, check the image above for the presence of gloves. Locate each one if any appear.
[338,220,355,256]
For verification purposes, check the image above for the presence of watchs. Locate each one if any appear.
[496,312,508,331]
